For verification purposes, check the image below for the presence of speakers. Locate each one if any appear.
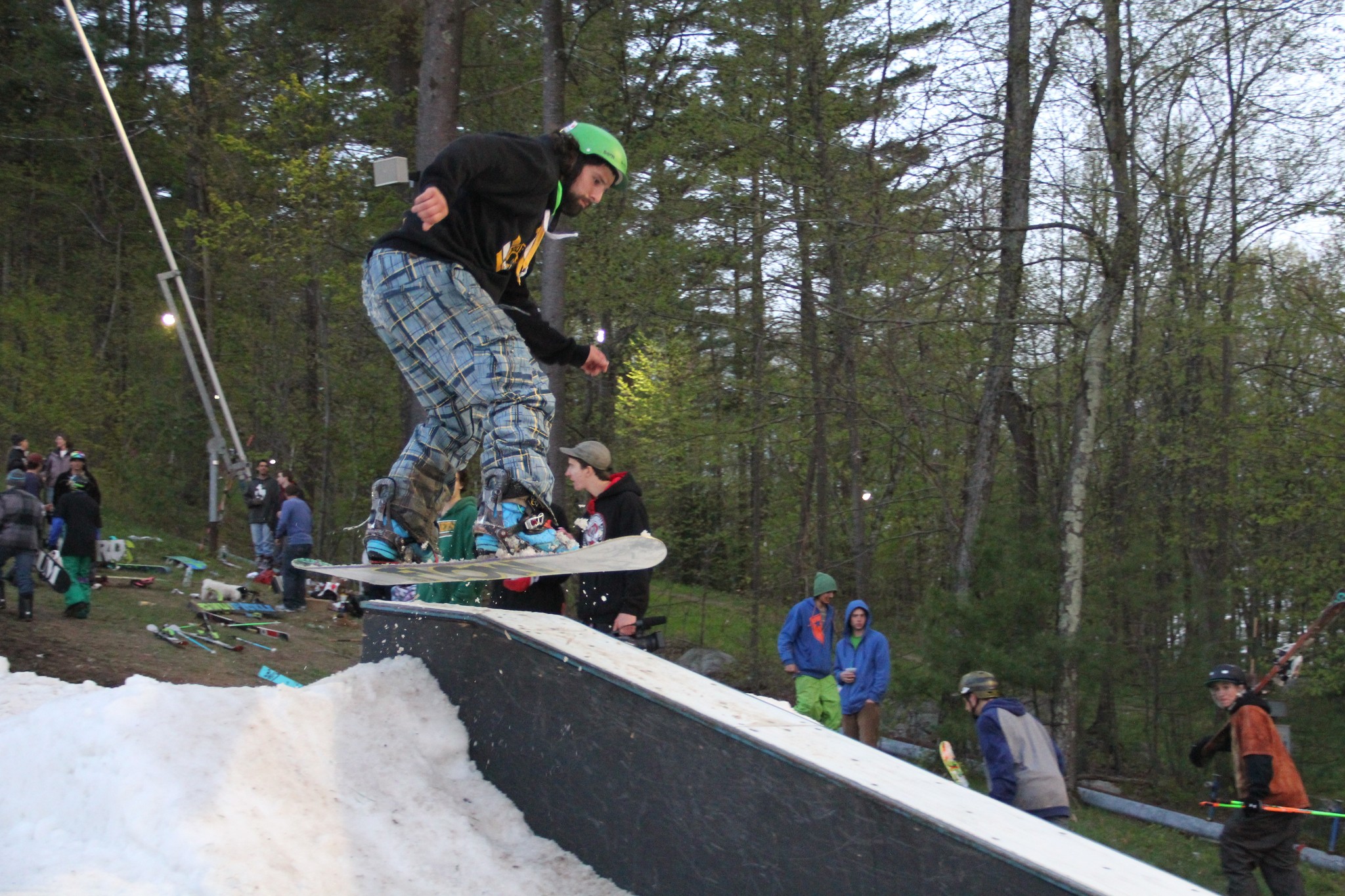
[373,156,409,187]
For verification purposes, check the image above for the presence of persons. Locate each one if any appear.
[473,483,571,614]
[832,599,890,748]
[951,671,1072,830]
[1188,664,1307,896]
[777,571,845,730]
[0,435,102,625]
[359,121,631,567]
[246,457,314,610]
[415,464,492,607]
[559,439,653,641]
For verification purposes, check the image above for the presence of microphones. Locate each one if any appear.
[636,617,667,630]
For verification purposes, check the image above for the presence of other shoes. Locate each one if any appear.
[365,516,443,565]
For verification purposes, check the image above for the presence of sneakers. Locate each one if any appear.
[275,604,294,612]
[65,602,87,618]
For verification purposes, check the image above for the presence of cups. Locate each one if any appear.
[845,667,857,682]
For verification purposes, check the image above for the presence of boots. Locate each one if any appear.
[18,593,33,620]
[0,580,5,608]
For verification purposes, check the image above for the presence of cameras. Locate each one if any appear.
[594,631,667,652]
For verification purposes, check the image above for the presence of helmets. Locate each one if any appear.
[559,121,627,186]
[950,670,1003,698]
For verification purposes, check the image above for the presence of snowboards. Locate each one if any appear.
[1189,585,1345,769]
[288,529,670,588]
[90,573,157,587]
[104,559,172,574]
[33,547,72,595]
[160,555,208,572]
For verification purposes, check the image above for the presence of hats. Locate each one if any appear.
[843,599,872,637]
[559,440,611,471]
[1202,664,1247,685]
[4,468,26,487]
[69,474,89,491]
[26,452,43,463]
[69,451,87,462]
[813,571,838,597]
[475,501,579,555]
[10,434,25,445]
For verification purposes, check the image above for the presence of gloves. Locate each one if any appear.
[1243,790,1262,814]
[1188,744,1212,766]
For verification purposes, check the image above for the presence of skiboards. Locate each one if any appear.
[147,601,292,656]
[936,739,970,790]
